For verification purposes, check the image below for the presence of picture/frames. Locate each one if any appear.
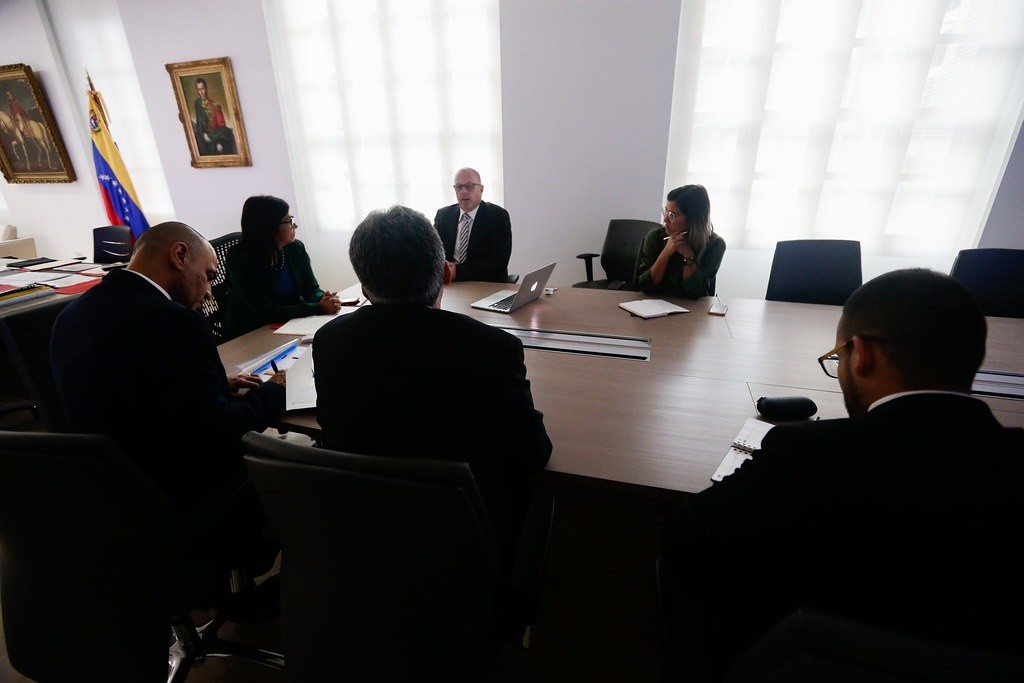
[0,62,77,183]
[164,56,251,168]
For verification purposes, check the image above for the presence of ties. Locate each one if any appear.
[458,213,470,263]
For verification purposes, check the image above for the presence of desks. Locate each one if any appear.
[214,279,1024,493]
[0,258,106,319]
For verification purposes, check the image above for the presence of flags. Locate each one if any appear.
[88,93,151,244]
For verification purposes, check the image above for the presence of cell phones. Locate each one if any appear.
[710,305,728,316]
[339,297,359,306]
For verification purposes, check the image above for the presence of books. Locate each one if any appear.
[619,299,690,319]
[0,284,54,306]
[711,418,775,482]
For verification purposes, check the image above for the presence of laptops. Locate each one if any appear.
[470,261,557,314]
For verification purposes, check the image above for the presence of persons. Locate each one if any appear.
[50,222,281,621]
[665,269,1024,682]
[638,185,726,300]
[434,167,512,282]
[223,195,341,340]
[312,206,553,646]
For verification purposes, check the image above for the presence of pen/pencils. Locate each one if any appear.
[662,232,688,241]
[270,359,278,372]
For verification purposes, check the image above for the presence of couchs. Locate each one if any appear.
[0,225,37,259]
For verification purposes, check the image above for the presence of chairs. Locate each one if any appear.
[241,431,555,683]
[93,225,133,264]
[0,429,286,683]
[195,231,244,342]
[722,605,1024,682]
[949,248,1024,320]
[571,219,663,291]
[766,240,862,307]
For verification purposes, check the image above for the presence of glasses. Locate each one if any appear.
[281,215,295,226]
[662,207,687,223]
[453,181,482,192]
[818,332,876,377]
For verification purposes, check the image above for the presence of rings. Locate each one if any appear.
[246,373,251,376]
